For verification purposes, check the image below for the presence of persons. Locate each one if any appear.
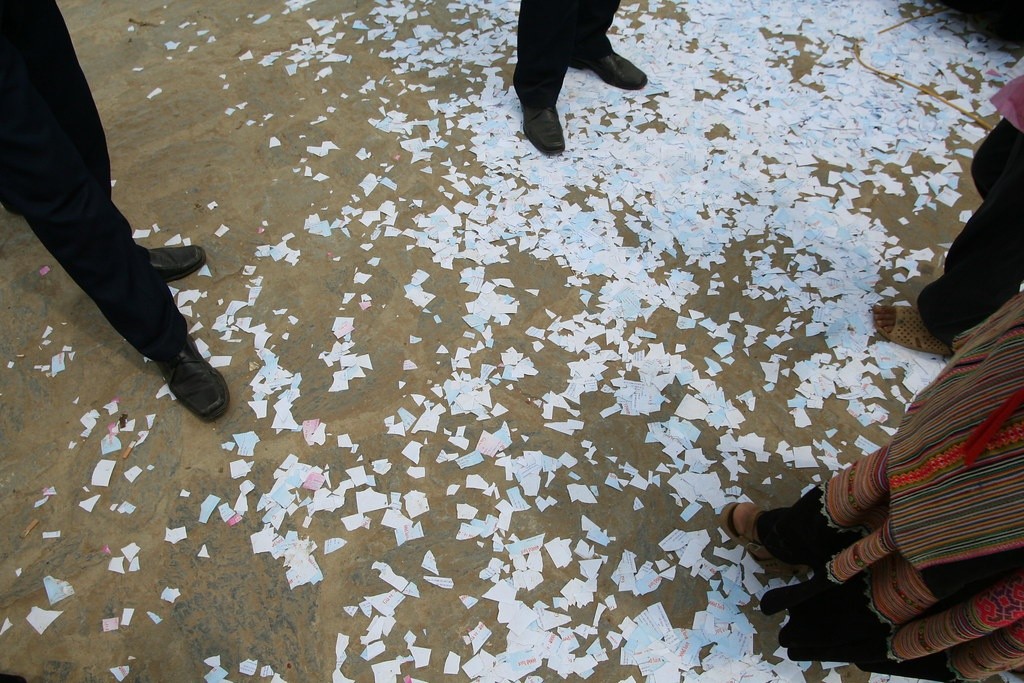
[0,0,230,420]
[720,291,1024,683]
[873,74,1024,357]
[512,0,648,154]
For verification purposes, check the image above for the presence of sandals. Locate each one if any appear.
[872,306,954,354]
[721,502,810,578]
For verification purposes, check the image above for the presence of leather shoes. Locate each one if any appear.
[522,103,565,152]
[147,245,205,281]
[573,51,648,90]
[156,332,231,420]
